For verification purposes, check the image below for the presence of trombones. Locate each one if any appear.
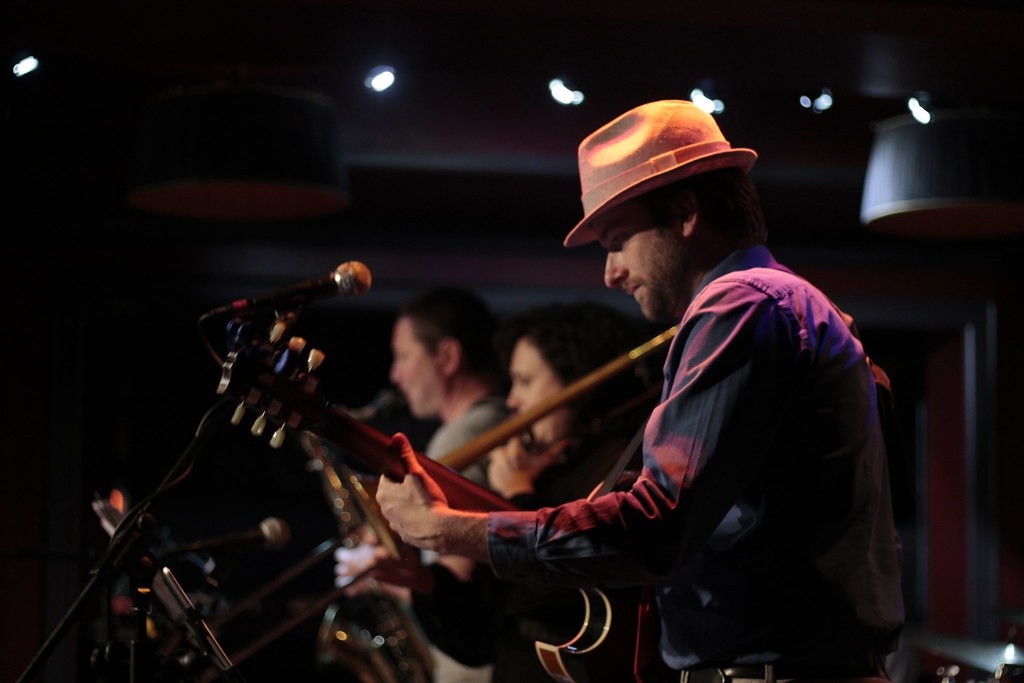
[162,323,680,683]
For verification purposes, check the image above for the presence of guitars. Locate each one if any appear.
[217,317,679,683]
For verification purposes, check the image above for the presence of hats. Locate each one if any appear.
[562,97,759,251]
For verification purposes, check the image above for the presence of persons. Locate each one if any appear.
[342,287,506,683]
[335,304,674,683]
[375,98,905,683]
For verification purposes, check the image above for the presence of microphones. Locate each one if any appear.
[212,260,370,317]
[156,516,291,559]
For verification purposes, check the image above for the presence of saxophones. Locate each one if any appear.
[295,392,435,683]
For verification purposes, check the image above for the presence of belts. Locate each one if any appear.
[680,651,886,683]
